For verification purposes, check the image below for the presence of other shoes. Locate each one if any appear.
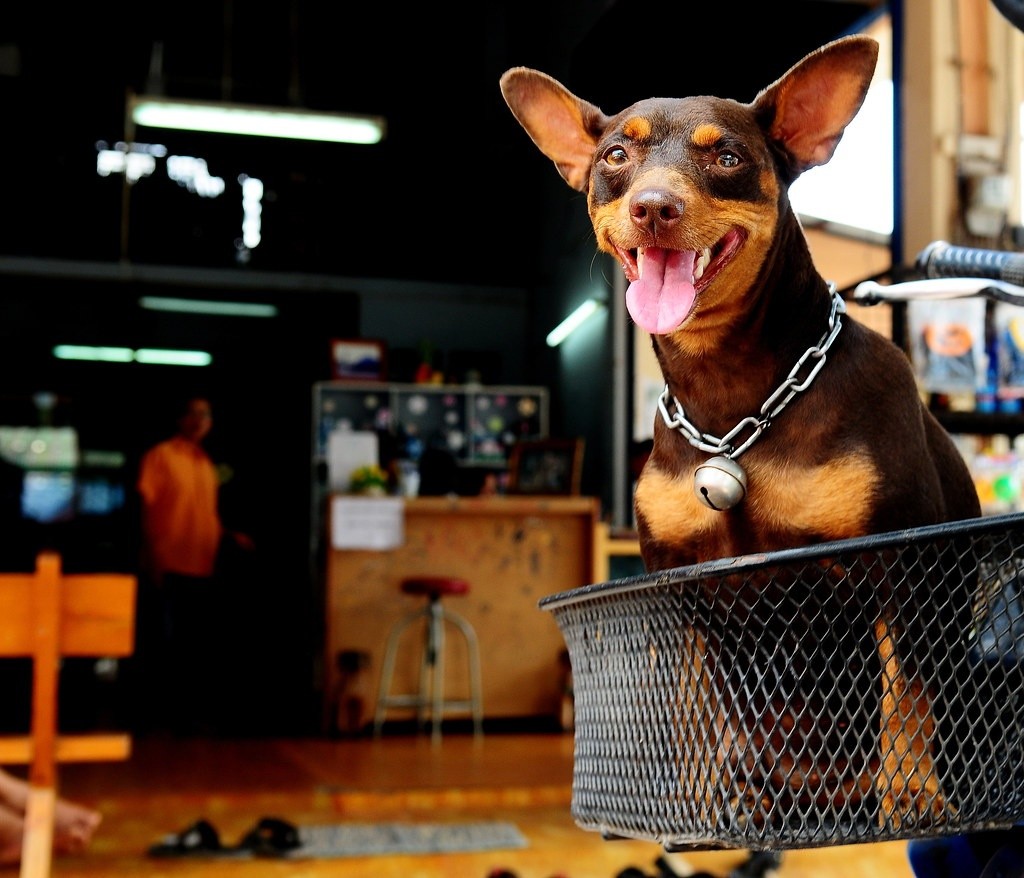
[231,816,303,860]
[147,819,229,860]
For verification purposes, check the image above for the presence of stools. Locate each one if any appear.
[373,575,488,749]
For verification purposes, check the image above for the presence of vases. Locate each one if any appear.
[390,460,423,497]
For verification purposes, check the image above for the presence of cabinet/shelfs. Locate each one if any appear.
[306,385,549,495]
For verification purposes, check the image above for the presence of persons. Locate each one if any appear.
[136,396,224,738]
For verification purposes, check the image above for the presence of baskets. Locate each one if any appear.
[536,509,1024,853]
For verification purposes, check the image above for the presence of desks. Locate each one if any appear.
[318,495,600,738]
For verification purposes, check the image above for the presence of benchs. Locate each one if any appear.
[0,555,137,878]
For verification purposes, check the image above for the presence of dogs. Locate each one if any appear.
[500,33,982,829]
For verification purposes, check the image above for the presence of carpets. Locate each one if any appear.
[288,823,529,862]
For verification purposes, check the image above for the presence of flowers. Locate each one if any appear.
[406,439,425,462]
